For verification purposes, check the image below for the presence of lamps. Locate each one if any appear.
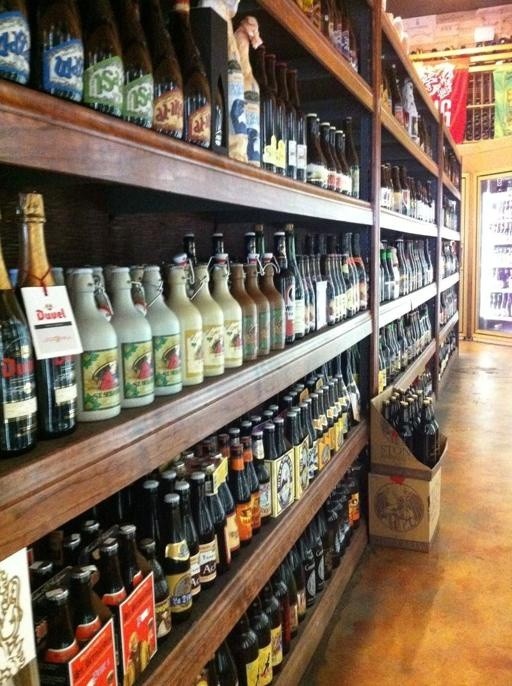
[473,27,495,47]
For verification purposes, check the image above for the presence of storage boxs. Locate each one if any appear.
[369,390,449,554]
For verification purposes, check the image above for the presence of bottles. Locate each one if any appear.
[477,178,512,335]
[461,39,512,65]
[462,96,494,142]
[2,0,460,686]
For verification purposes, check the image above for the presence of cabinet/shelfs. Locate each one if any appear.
[0,1,461,685]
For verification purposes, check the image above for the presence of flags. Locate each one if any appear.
[412,57,470,144]
